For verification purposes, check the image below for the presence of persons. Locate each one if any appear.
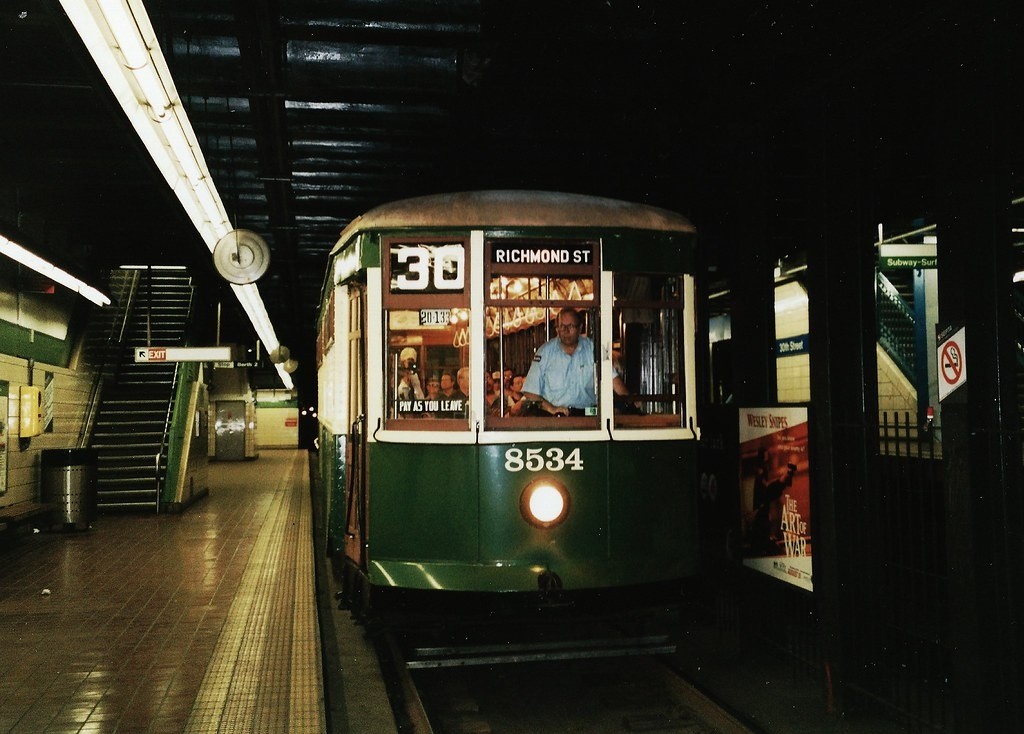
[519,308,642,417]
[397,358,525,419]
[612,341,624,374]
[749,437,795,557]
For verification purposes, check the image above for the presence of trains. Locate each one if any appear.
[316,189,704,634]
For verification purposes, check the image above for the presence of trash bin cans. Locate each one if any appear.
[39,446,99,532]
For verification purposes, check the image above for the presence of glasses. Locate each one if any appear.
[558,324,578,331]
